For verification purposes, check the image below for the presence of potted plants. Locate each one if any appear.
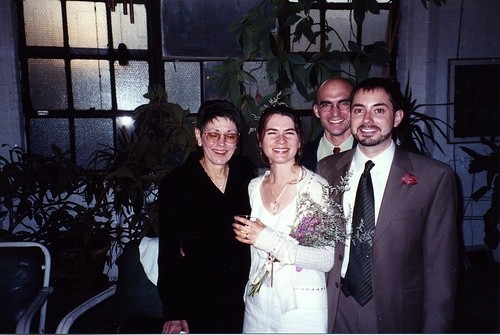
[0,133,167,290]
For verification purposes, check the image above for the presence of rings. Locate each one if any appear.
[246,233,248,239]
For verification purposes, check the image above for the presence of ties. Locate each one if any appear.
[341,161,375,306]
[333,148,340,154]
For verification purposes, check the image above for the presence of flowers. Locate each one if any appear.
[250,179,374,296]
[402,173,421,190]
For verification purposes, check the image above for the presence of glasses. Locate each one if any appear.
[315,99,350,112]
[201,129,238,143]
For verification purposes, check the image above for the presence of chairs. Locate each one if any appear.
[56,238,165,334]
[0,241,54,334]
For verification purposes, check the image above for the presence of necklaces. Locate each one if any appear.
[268,182,289,214]
[211,179,226,189]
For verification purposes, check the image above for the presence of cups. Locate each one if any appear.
[235,215,258,240]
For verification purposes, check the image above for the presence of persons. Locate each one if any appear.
[233,103,335,335]
[299,76,358,174]
[315,78,459,335]
[158,99,257,335]
